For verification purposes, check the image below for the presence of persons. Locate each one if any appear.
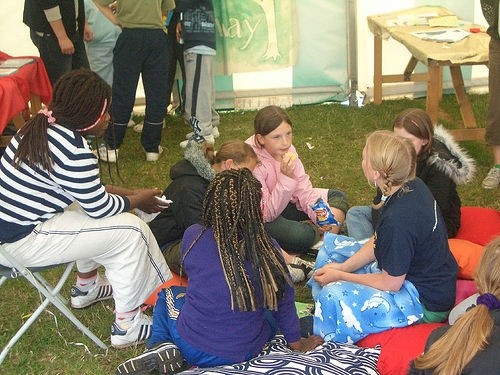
[112,166,325,374]
[143,139,317,286]
[302,129,462,347]
[93,0,175,162]
[173,0,222,148]
[22,0,89,82]
[345,106,475,244]
[83,0,138,129]
[480,0,499,193]
[407,237,500,375]
[245,104,349,260]
[0,67,173,348]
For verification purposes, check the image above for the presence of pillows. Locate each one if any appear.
[444,238,487,281]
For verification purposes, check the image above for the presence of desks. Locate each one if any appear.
[366,5,490,144]
[0,50,54,153]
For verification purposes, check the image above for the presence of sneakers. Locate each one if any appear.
[286,256,316,284]
[110,314,155,350]
[146,145,164,160]
[71,278,112,308]
[134,118,166,133]
[180,135,214,147]
[187,126,221,139]
[92,147,120,163]
[116,342,184,375]
[484,166,500,189]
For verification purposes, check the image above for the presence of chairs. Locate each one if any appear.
[0,245,105,365]
